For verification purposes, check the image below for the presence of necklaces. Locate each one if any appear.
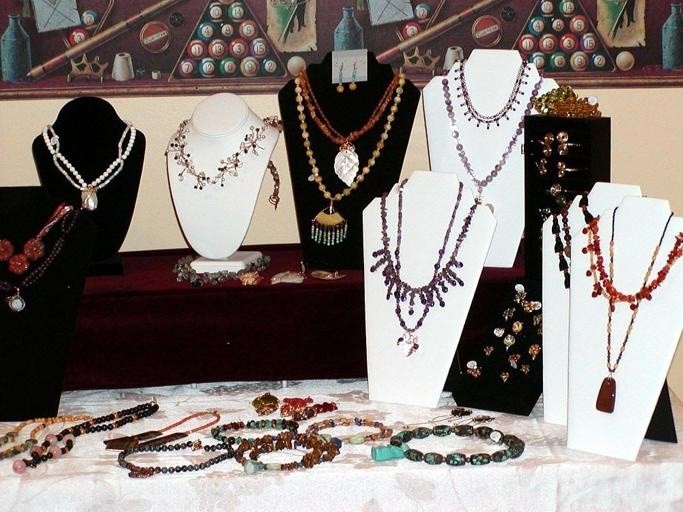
[444,74,537,215]
[545,186,598,290]
[163,116,285,213]
[1,386,538,480]
[597,202,676,430]
[457,58,533,133]
[43,123,135,214]
[0,205,79,314]
[396,175,463,354]
[171,253,277,288]
[371,193,489,352]
[294,69,410,244]
[595,210,683,300]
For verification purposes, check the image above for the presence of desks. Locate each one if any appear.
[69,240,541,415]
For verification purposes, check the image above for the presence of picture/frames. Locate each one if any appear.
[0,1,683,98]
